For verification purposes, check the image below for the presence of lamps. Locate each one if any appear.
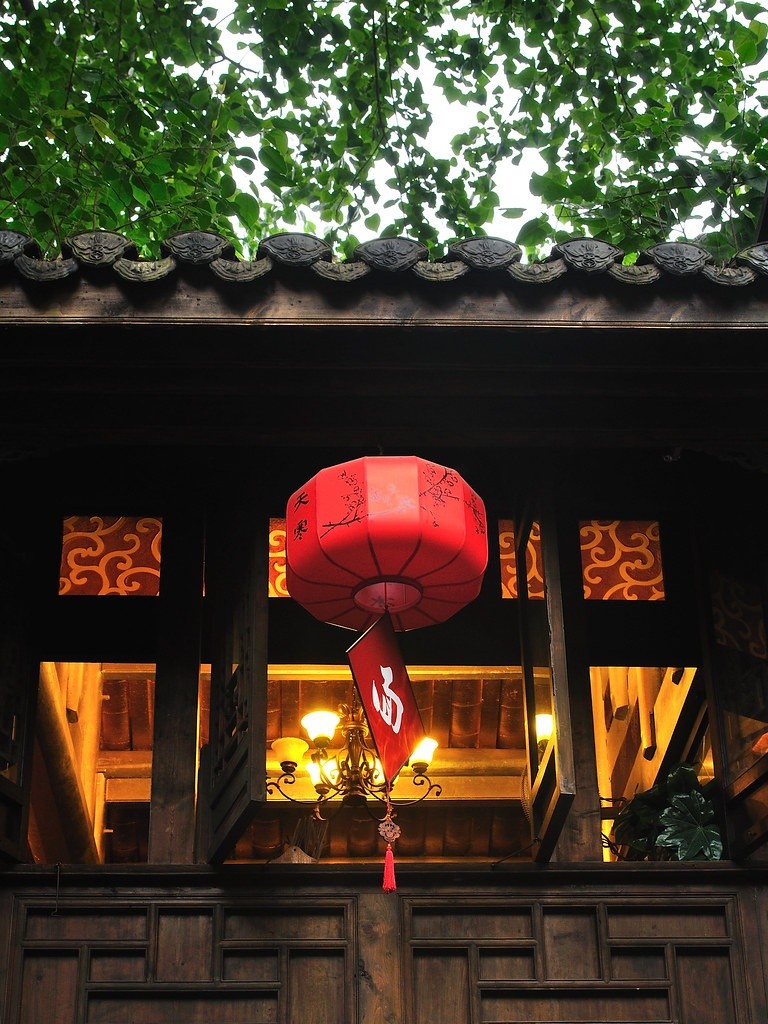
[535,713,556,764]
[264,681,442,823]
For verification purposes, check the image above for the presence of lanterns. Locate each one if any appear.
[287,455,490,893]
